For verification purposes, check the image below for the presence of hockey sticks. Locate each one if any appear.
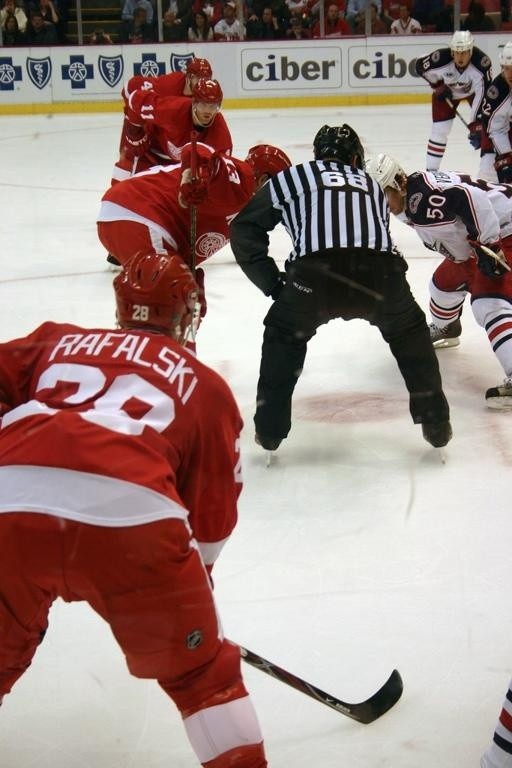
[224,637,403,723]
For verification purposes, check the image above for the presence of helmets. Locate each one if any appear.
[498,39,512,66]
[314,123,364,171]
[365,153,407,193]
[244,144,292,179]
[451,30,474,57]
[187,58,222,106]
[113,248,201,358]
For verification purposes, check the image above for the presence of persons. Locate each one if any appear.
[1,247,268,767]
[479,677,512,768]
[1,1,512,45]
[363,153,512,400]
[121,57,212,115]
[106,76,232,266]
[96,140,293,357]
[477,41,512,186]
[229,124,453,451]
[415,30,498,183]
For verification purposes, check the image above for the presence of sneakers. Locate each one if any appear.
[486,378,512,397]
[422,418,452,446]
[429,318,462,342]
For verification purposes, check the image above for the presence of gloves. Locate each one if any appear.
[494,151,512,183]
[125,129,151,157]
[468,121,484,148]
[430,76,452,103]
[180,166,207,203]
[466,234,508,279]
